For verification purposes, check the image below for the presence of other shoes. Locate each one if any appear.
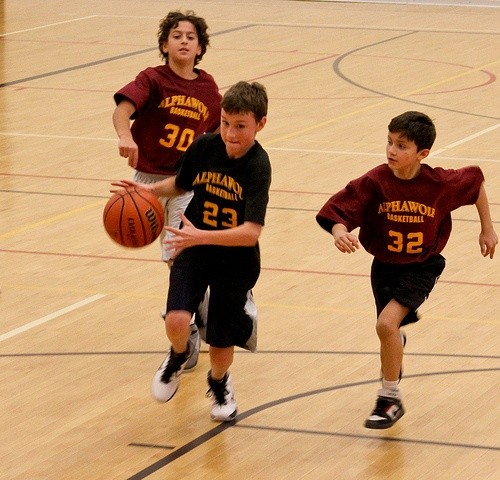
[184,332,200,369]
[195,291,209,340]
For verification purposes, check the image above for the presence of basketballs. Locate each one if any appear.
[103,187,165,247]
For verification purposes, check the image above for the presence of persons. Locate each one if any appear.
[112,10,257,352]
[110,81,271,421]
[316,111,497,430]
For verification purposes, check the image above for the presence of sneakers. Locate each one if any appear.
[207,367,237,422]
[379,333,407,388]
[364,397,406,429]
[150,337,196,403]
